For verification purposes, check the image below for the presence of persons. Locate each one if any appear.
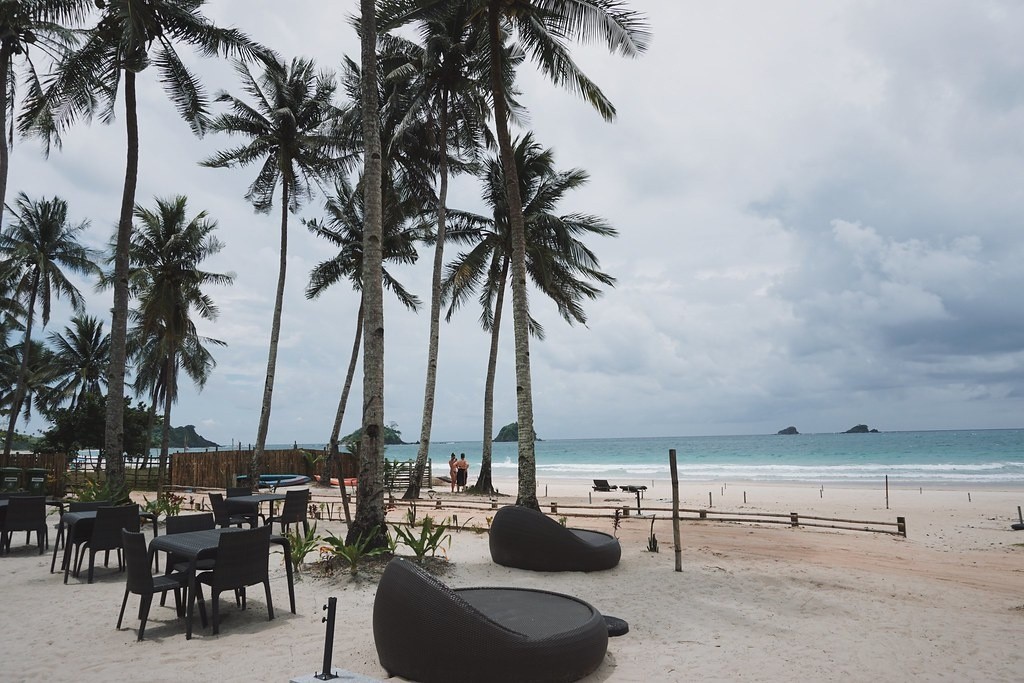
[449,452,469,493]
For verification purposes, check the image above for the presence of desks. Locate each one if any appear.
[139,526,297,641]
[0,500,65,550]
[50,511,159,586]
[225,493,311,538]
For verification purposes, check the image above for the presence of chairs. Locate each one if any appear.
[0,485,315,642]
[591,479,618,492]
[371,555,610,682]
[619,484,648,493]
[488,503,623,572]
